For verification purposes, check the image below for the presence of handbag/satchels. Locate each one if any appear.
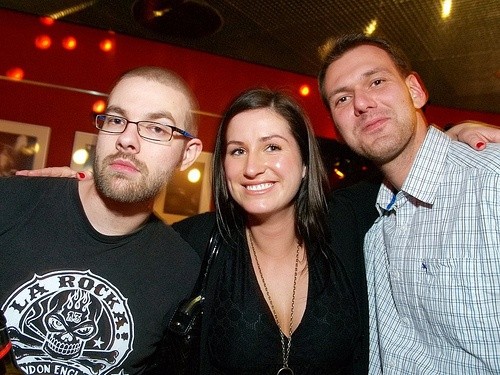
[161,225,226,375]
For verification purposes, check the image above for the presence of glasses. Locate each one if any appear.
[96,114,197,142]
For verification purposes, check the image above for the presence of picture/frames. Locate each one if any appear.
[154,149,220,225]
[69,130,99,173]
[0,118,52,179]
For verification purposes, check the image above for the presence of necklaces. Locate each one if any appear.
[248,226,300,375]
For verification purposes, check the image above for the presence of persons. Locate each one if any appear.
[0,66,203,375]
[15,87,500,375]
[318,34,500,375]
